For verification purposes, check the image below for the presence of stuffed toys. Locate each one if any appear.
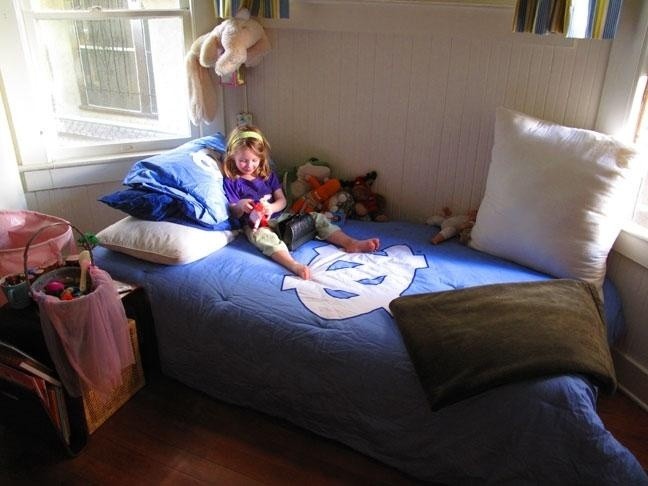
[186,7,273,128]
[274,155,387,225]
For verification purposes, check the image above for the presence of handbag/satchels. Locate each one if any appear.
[275,211,316,252]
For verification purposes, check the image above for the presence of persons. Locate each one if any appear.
[221,121,381,280]
[424,205,480,246]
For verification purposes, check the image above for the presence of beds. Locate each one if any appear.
[86,212,620,483]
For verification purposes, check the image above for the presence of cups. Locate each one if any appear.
[0,272,30,311]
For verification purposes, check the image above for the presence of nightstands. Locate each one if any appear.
[0,271,162,460]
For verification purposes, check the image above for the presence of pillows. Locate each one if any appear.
[92,189,242,266]
[466,104,638,293]
